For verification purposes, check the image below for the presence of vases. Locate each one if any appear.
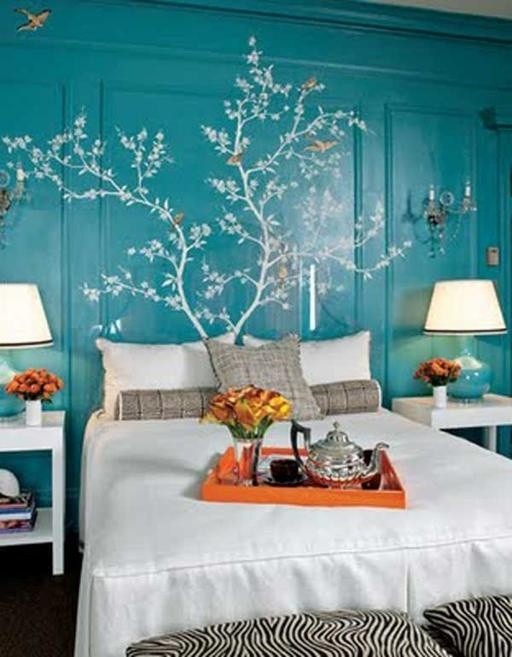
[230,438,263,488]
[433,382,447,408]
[25,400,41,426]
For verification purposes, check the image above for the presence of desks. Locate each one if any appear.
[0,409,67,575]
[393,391,512,462]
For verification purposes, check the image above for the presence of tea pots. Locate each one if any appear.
[290,417,389,489]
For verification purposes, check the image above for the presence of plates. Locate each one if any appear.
[261,475,305,486]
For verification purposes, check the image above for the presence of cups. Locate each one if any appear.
[272,458,299,481]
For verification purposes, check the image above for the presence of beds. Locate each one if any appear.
[72,403,512,656]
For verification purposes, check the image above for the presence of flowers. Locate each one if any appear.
[202,384,292,438]
[413,356,463,388]
[3,368,66,404]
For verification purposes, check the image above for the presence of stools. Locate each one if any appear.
[125,605,456,657]
[422,590,512,657]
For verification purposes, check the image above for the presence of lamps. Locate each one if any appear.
[420,174,477,264]
[423,276,508,402]
[1,154,28,247]
[1,282,55,421]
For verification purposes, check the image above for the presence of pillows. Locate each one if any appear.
[112,386,219,422]
[240,330,371,385]
[202,334,326,424]
[95,334,222,420]
[312,377,381,413]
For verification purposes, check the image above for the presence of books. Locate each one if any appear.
[1,488,38,534]
